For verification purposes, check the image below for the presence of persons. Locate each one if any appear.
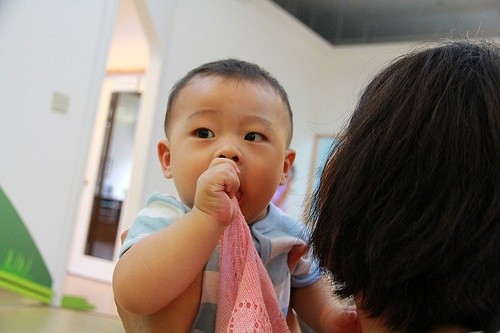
[113,25,499,332]
[113,59,361,333]
